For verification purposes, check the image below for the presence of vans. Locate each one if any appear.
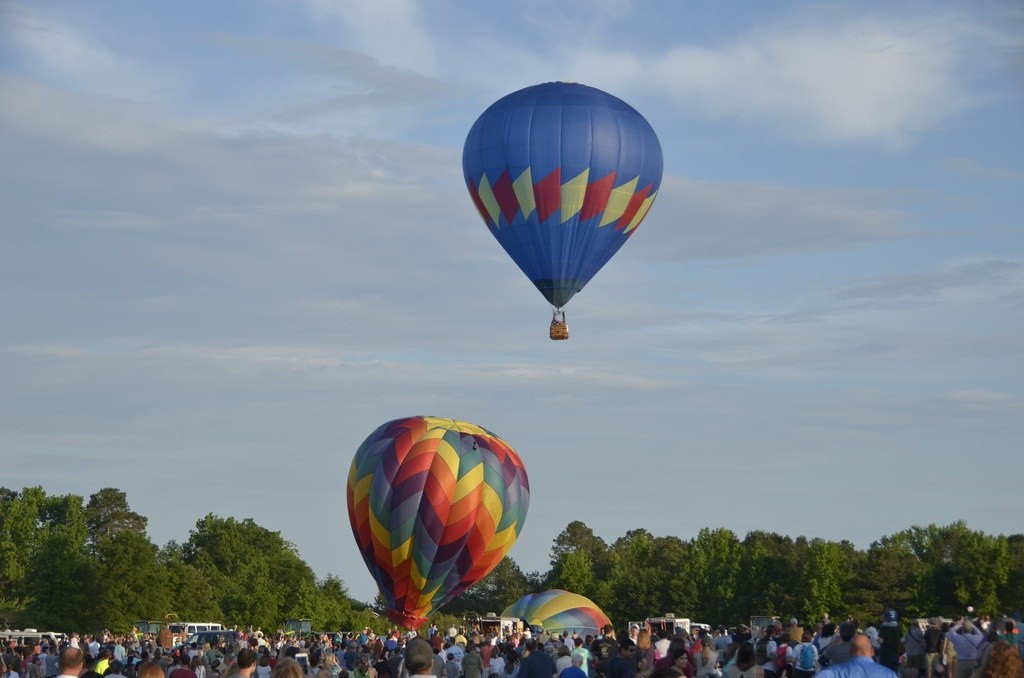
[168,622,227,637]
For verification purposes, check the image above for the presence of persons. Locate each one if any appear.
[0,612,1024,678]
[815,634,897,678]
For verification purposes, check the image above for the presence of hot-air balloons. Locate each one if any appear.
[463,83,663,341]
[347,413,531,629]
[500,588,616,646]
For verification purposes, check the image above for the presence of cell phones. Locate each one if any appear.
[295,654,309,675]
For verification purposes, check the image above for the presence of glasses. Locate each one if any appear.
[184,648,187,649]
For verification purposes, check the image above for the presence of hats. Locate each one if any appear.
[791,618,797,624]
[552,633,559,640]
[927,617,939,626]
[405,639,433,670]
[630,624,639,630]
[963,620,972,629]
[1003,618,1015,627]
